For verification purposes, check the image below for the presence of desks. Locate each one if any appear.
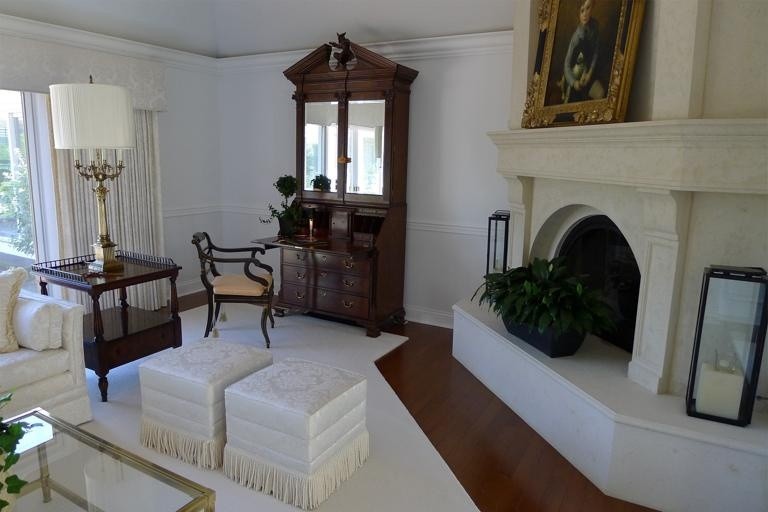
[32,252,184,402]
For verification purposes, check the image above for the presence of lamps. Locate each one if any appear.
[49,74,136,276]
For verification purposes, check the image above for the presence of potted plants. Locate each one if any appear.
[258,174,317,239]
[471,257,626,358]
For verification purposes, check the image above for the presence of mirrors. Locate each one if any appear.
[303,101,338,191]
[345,100,385,196]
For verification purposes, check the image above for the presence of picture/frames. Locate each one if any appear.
[521,0,647,129]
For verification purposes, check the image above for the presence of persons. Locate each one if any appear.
[562,1,607,101]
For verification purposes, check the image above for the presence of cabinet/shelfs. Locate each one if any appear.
[292,92,410,208]
[273,244,406,338]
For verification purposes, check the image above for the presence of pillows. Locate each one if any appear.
[0,266,29,353]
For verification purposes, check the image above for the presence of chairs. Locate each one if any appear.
[191,232,275,349]
[0,294,94,431]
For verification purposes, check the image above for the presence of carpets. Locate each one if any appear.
[0,302,480,512]
